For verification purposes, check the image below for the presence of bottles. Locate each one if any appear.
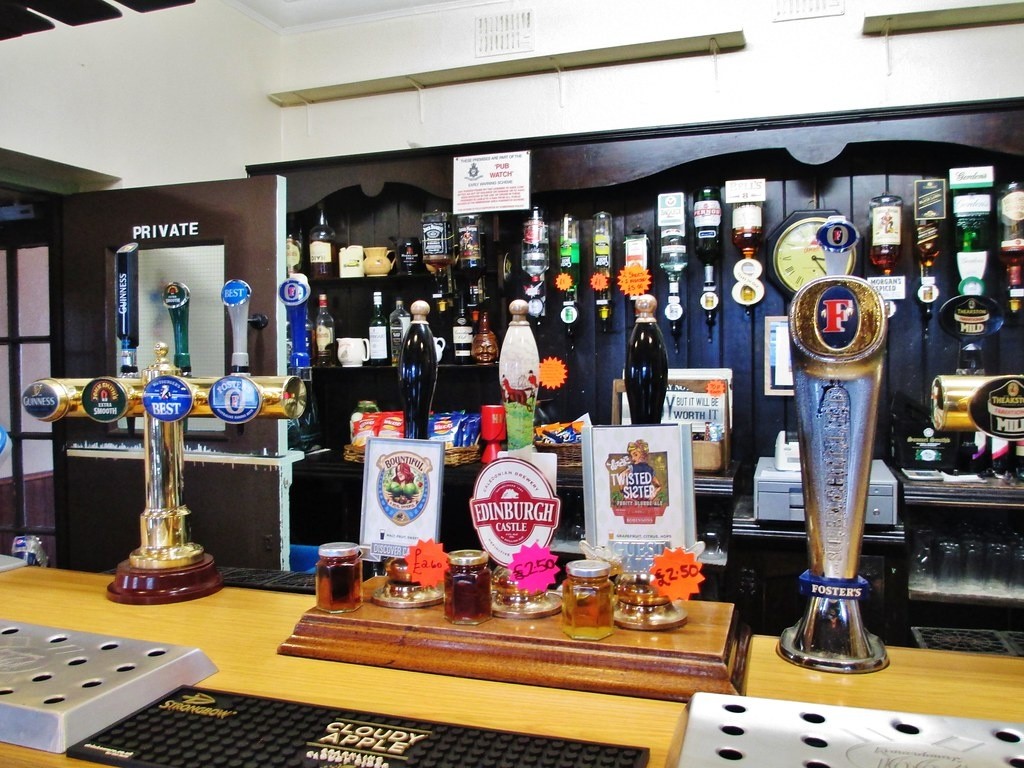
[949,165,997,307]
[338,245,365,278]
[996,181,1024,313]
[443,550,493,625]
[624,227,653,330]
[913,178,946,338]
[285,212,306,278]
[368,291,389,366]
[866,195,903,317]
[315,542,365,614]
[692,186,721,342]
[450,290,478,365]
[308,203,339,281]
[560,560,614,640]
[315,294,337,367]
[456,214,484,325]
[591,211,614,331]
[522,206,548,324]
[558,213,580,332]
[420,211,454,317]
[389,297,410,365]
[302,299,316,368]
[657,192,686,355]
[730,179,765,315]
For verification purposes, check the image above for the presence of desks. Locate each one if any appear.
[1,565,1024,768]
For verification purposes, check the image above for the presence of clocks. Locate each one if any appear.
[764,208,865,301]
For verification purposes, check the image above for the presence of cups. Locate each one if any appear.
[363,247,396,276]
[389,236,422,277]
[432,337,445,364]
[336,338,370,368]
